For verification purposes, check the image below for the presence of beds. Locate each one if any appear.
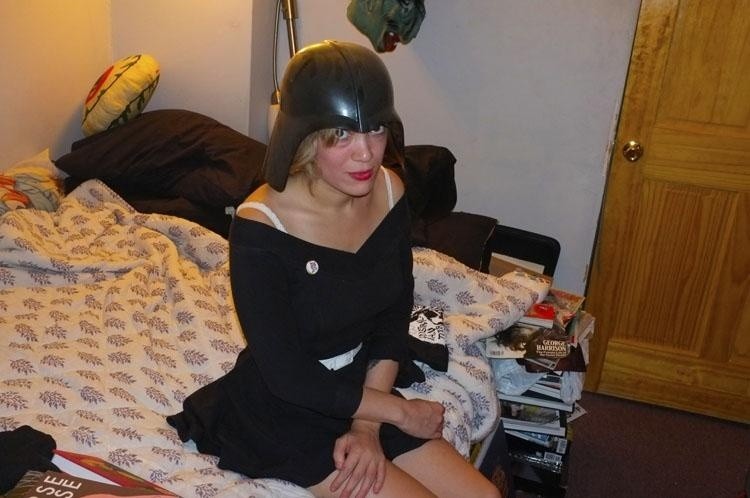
[1,151,560,498]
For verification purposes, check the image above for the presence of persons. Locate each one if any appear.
[164,38,503,497]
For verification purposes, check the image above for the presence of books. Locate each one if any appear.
[50,448,184,497]
[3,469,161,496]
[477,251,598,496]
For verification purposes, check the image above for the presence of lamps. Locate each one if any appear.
[268,0,298,139]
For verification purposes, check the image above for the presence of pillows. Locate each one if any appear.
[53,105,269,240]
[403,146,456,246]
[79,53,160,139]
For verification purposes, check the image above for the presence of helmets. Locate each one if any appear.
[260,39,406,193]
